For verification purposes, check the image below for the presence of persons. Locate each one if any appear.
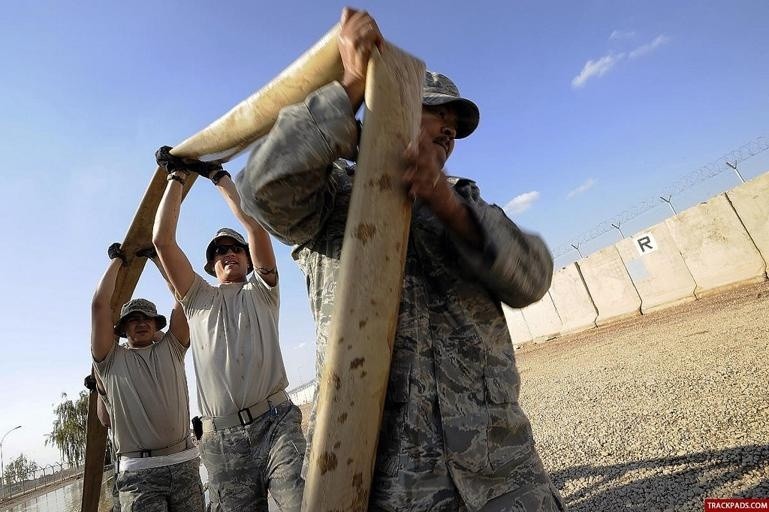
[149,144,305,512]
[89,242,207,512]
[96,394,121,512]
[232,4,567,512]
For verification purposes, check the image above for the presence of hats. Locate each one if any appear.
[203,228,254,278]
[422,70,480,139]
[114,298,167,338]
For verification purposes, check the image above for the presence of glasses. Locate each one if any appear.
[214,244,244,256]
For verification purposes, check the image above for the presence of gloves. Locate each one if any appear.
[107,242,127,265]
[84,375,96,391]
[135,246,157,258]
[155,145,228,179]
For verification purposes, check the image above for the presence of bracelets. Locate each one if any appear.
[211,170,231,185]
[166,174,185,186]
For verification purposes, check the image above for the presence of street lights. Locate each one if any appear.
[571,242,583,258]
[659,195,677,215]
[39,461,64,487]
[725,159,747,183]
[611,220,625,239]
[0,426,22,501]
